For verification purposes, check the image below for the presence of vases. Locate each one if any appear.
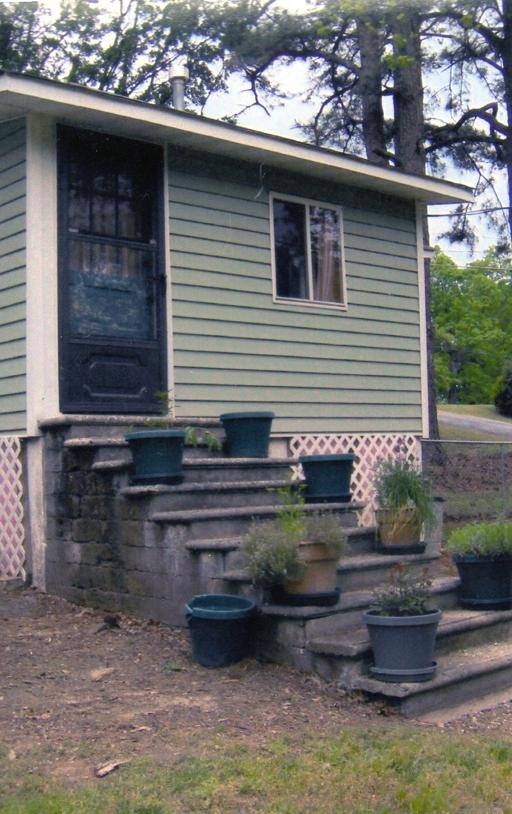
[221,409,356,502]
[183,592,256,668]
[122,387,224,482]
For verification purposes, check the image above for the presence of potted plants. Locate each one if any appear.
[446,523,511,609]
[362,562,442,681]
[236,533,295,604]
[265,467,344,605]
[375,463,432,552]
